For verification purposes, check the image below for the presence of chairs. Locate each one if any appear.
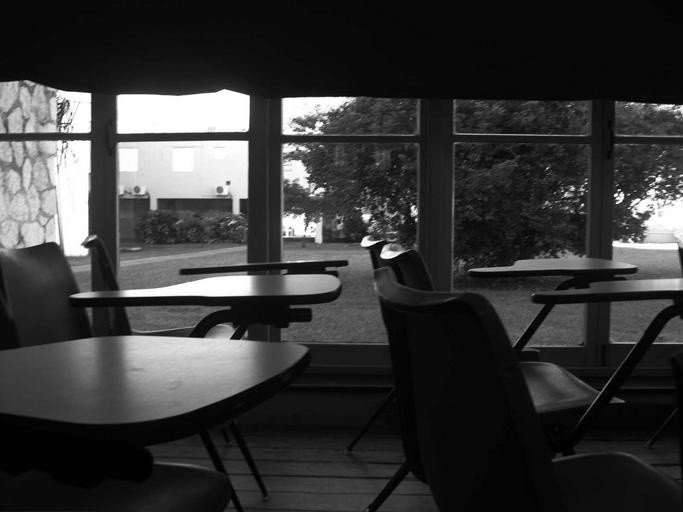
[371,267,683,512]
[0,463,235,512]
[359,228,638,510]
[0,233,348,381]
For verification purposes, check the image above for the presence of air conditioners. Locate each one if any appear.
[131,185,145,195]
[215,185,228,197]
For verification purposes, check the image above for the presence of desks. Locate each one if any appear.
[525,279,683,450]
[3,333,311,510]
